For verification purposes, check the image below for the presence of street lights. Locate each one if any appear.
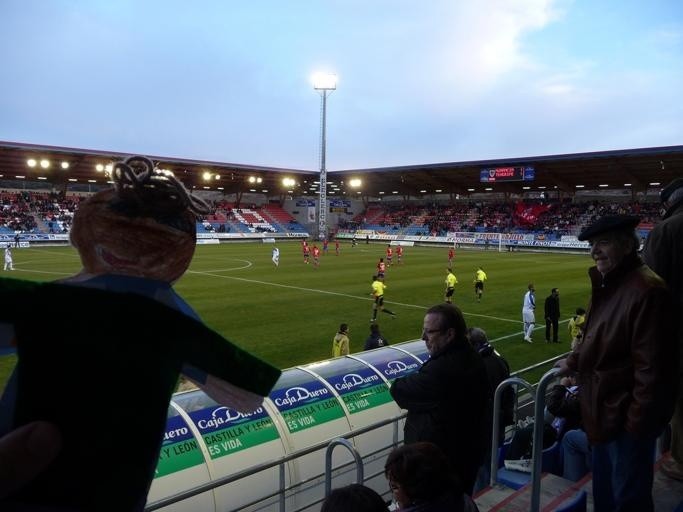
[313,72,336,241]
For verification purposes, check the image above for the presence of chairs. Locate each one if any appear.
[0,190,309,238]
[335,203,660,242]
[494,384,672,511]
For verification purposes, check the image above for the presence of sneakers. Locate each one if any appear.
[658,459,683,480]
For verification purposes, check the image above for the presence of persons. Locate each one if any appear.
[514,374,616,483]
[522,284,538,343]
[0,419,61,501]
[448,249,454,265]
[340,197,663,237]
[2,245,14,271]
[318,482,392,511]
[330,323,349,357]
[567,307,587,351]
[299,237,339,266]
[388,302,490,501]
[362,323,389,351]
[641,177,682,481]
[14,234,20,248]
[375,257,385,282]
[380,439,479,511]
[197,195,296,234]
[395,244,402,264]
[0,188,96,234]
[271,246,279,272]
[444,267,458,304]
[474,266,487,302]
[544,287,561,345]
[460,325,517,469]
[369,275,396,322]
[385,244,393,267]
[552,211,683,511]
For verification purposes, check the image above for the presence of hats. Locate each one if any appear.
[578,215,641,241]
[661,178,683,202]
